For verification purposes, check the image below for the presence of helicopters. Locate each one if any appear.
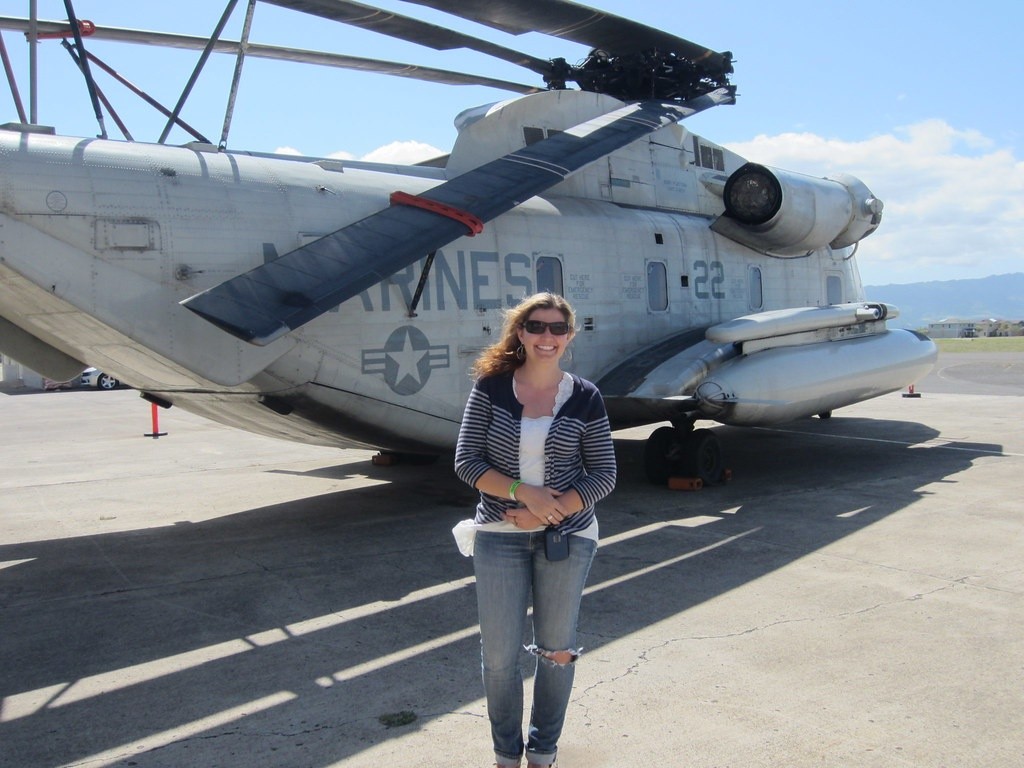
[0,0,938,462]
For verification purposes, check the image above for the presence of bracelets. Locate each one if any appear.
[510,480,525,503]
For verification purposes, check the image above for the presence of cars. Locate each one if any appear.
[81,367,127,390]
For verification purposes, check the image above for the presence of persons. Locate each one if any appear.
[454,291,617,768]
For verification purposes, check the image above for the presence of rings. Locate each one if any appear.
[547,515,553,520]
[513,517,518,526]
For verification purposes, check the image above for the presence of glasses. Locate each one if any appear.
[521,321,570,336]
